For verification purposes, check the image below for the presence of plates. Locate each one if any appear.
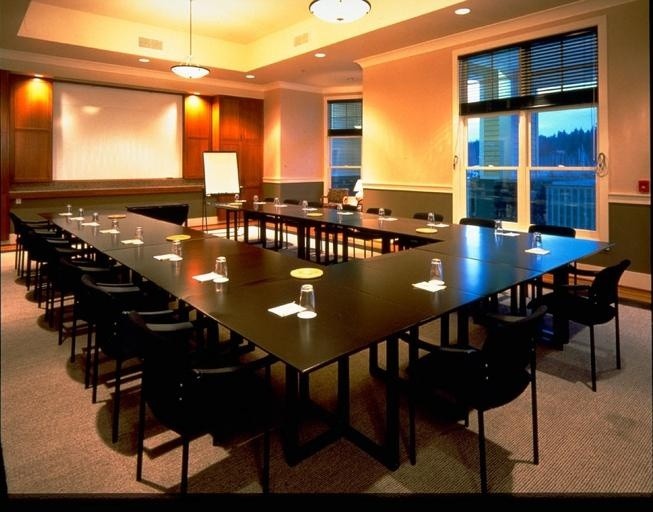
[290,266,324,279]
[166,234,191,241]
[307,212,323,217]
[415,227,438,234]
[108,214,126,218]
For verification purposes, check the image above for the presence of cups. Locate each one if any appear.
[92,212,99,222]
[214,256,228,279]
[234,194,239,200]
[302,200,308,209]
[532,232,542,248]
[254,195,258,202]
[336,203,343,212]
[67,205,72,213]
[274,198,280,205]
[298,284,316,313]
[111,219,119,232]
[494,219,502,231]
[78,208,83,217]
[135,227,144,242]
[430,258,444,282]
[171,240,182,257]
[378,208,385,217]
[427,212,434,223]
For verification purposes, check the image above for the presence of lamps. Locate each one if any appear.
[309,1,371,23]
[171,0,210,79]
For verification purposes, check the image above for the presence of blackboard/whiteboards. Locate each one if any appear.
[203,152,240,194]
